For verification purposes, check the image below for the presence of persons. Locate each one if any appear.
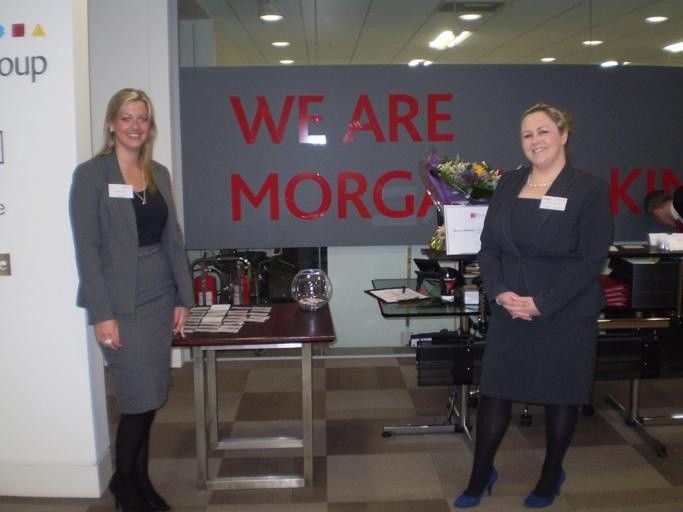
[70,88,197,512]
[454,104,614,507]
[643,185,683,228]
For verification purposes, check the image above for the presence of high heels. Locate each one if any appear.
[455,465,497,508]
[524,468,565,508]
[109,473,170,511]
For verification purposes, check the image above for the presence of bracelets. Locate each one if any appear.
[495,295,501,307]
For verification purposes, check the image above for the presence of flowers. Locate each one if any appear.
[425,151,506,251]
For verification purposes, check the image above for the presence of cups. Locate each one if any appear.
[441,277,458,303]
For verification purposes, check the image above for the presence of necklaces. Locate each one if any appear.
[116,156,148,205]
[526,173,552,187]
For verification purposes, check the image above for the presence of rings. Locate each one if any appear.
[105,338,112,344]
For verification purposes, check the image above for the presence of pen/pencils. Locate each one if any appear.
[403,285,406,293]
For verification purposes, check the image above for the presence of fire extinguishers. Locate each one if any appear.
[189,257,218,307]
[229,256,252,306]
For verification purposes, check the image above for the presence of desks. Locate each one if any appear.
[372,277,679,459]
[173,300,334,487]
